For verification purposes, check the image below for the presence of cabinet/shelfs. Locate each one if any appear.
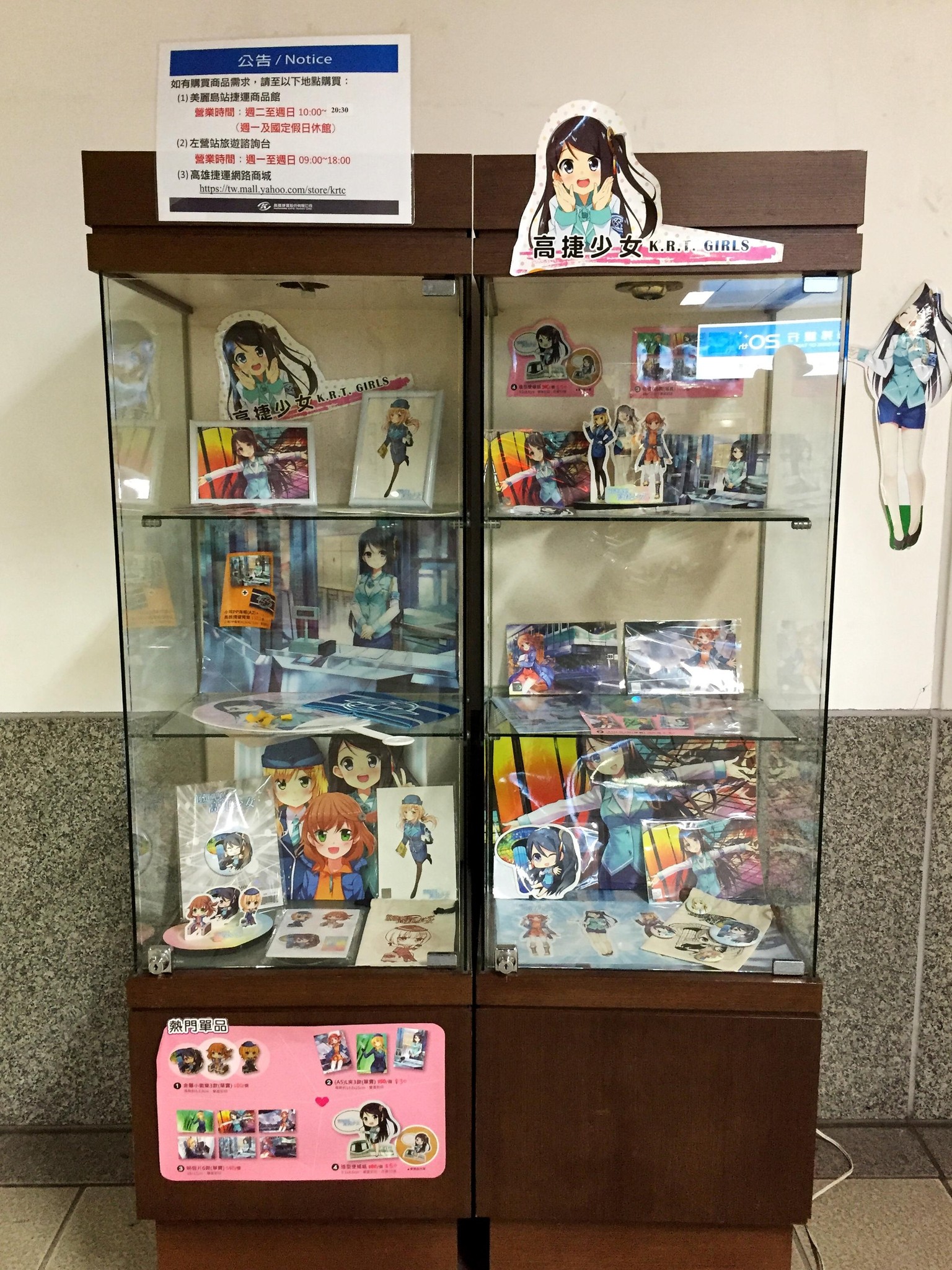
[79,150,868,1270]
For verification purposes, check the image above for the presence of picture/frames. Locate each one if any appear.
[348,389,444,509]
[188,418,320,508]
